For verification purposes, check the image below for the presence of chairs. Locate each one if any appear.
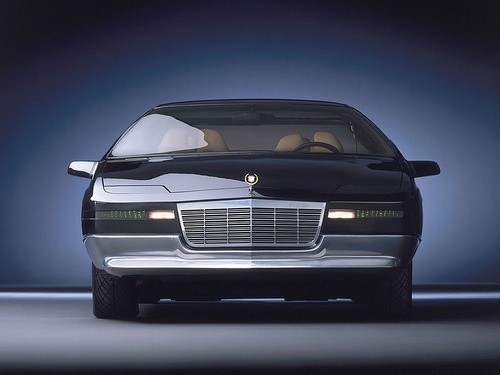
[157,128,229,152]
[274,131,344,153]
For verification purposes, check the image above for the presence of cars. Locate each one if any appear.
[67,98,441,321]
